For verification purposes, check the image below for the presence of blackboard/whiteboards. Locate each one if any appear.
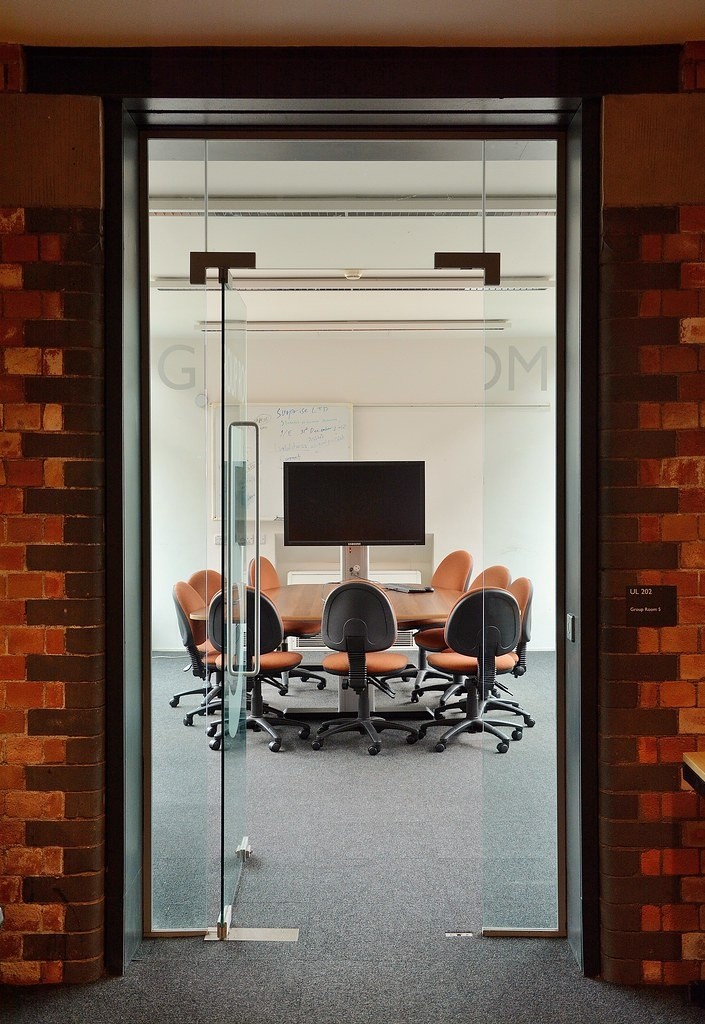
[212,403,353,522]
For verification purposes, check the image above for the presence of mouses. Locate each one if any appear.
[425,587,433,592]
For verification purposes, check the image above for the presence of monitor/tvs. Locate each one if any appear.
[283,461,424,547]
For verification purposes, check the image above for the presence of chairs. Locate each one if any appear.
[168,549,536,754]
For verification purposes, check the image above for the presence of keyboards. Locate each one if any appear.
[384,584,425,594]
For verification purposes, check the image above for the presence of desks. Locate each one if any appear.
[188,584,522,720]
